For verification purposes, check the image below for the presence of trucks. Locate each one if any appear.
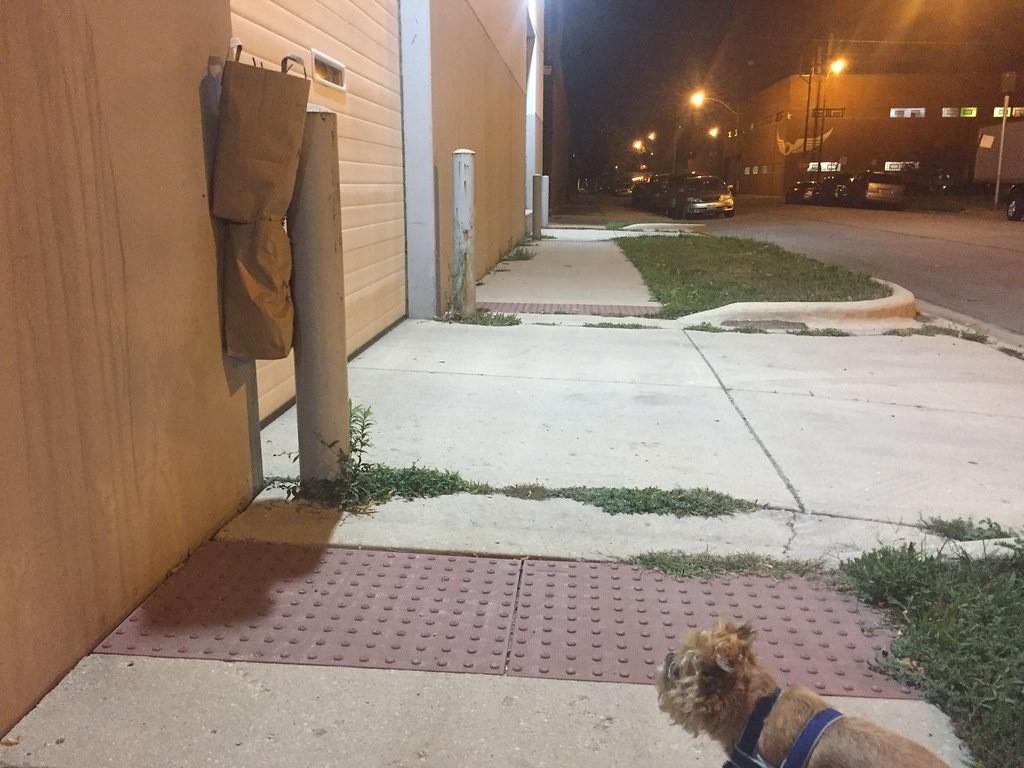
[971,120,1024,221]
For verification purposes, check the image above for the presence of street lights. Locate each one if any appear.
[816,59,845,181]
[689,90,740,197]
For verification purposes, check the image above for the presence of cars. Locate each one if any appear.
[594,169,736,220]
[784,170,914,210]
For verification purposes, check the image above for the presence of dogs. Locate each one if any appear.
[653,617,953,768]
